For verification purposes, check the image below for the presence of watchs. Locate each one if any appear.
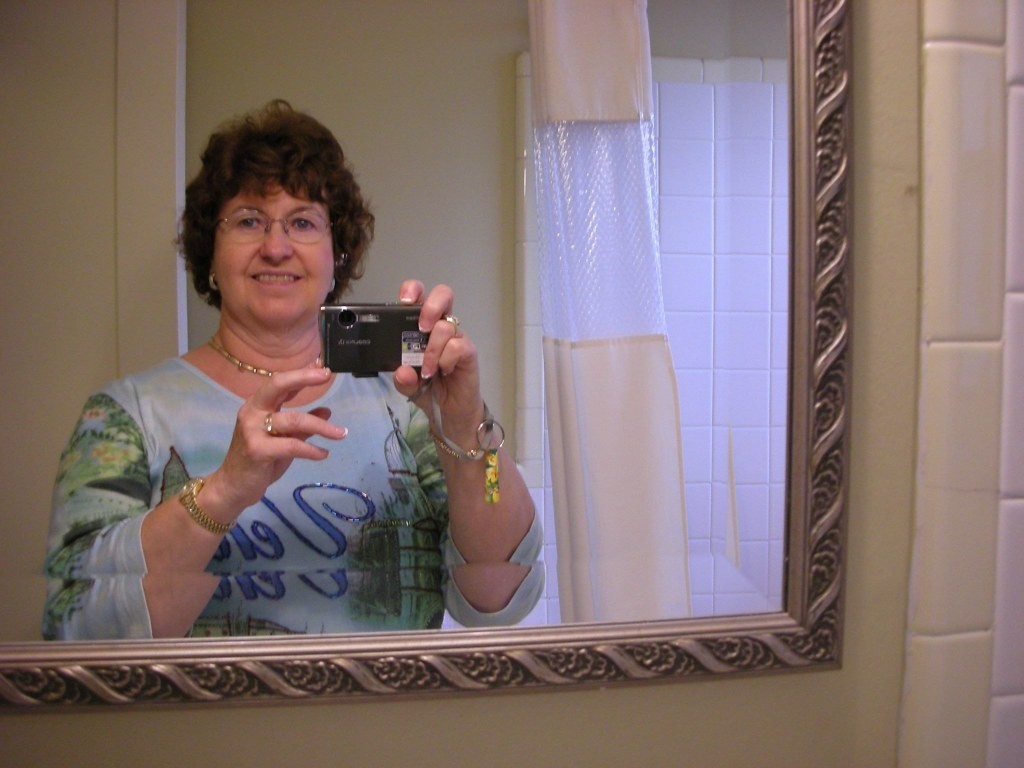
[180,477,239,535]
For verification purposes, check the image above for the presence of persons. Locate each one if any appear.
[40,95,545,569]
[41,563,544,640]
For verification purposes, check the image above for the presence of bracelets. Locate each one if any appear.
[424,429,478,466]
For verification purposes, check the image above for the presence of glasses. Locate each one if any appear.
[213,208,334,244]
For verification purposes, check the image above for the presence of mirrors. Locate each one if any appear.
[0,0,854,709]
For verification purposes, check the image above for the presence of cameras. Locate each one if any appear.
[319,301,438,378]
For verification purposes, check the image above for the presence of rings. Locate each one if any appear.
[264,412,278,438]
[441,312,463,337]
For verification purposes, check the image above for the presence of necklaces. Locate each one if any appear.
[205,338,324,381]
